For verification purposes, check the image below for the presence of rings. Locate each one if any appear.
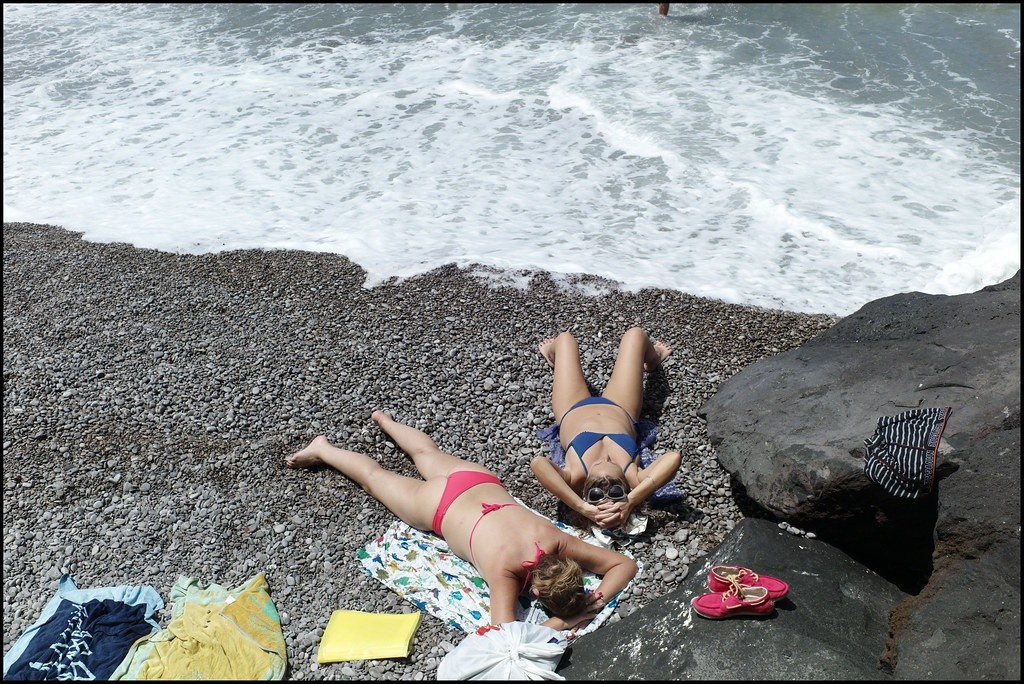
[582,601,588,606]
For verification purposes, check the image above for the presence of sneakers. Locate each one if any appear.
[692,566,789,619]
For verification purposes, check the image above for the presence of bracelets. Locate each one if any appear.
[647,474,658,491]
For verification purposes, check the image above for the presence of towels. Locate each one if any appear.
[2,574,287,680]
[535,417,690,522]
[356,492,646,653]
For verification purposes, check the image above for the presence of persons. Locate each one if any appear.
[529,327,681,530]
[286,409,639,631]
